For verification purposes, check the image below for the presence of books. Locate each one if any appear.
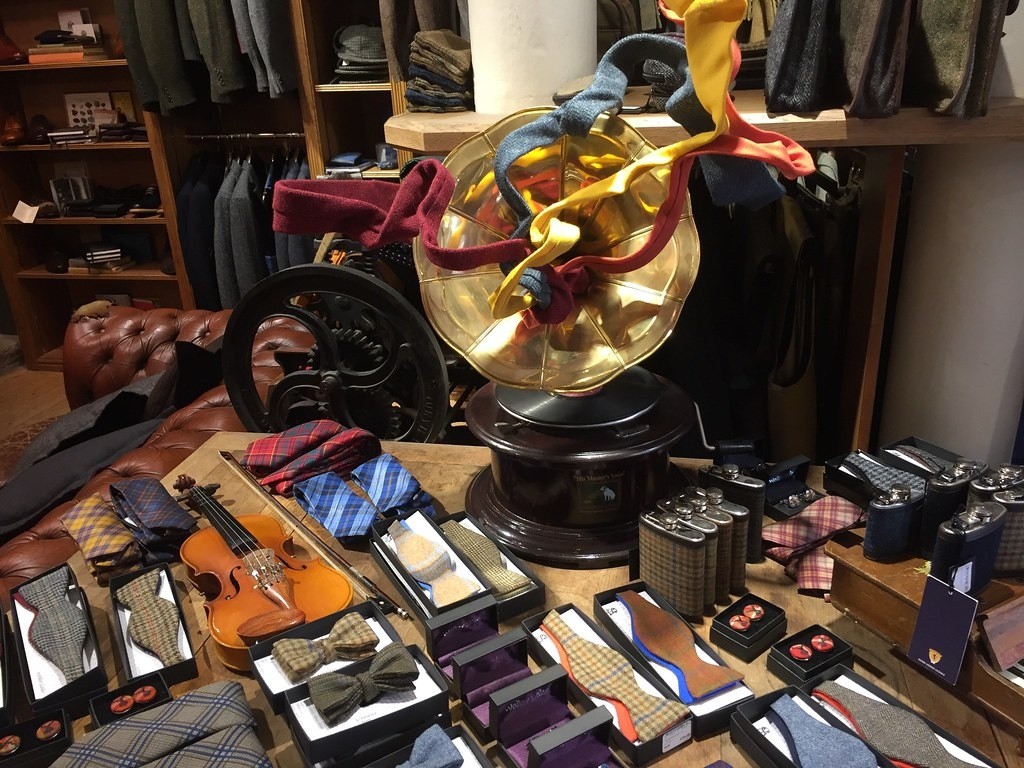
[323,153,375,173]
[29,44,107,62]
[47,123,92,144]
[68,245,135,274]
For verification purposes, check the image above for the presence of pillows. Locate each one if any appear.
[142,340,223,422]
[0,418,164,547]
[0,368,167,491]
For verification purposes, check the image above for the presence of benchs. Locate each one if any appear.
[0,300,317,629]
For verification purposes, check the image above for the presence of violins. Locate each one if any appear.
[169,473,356,672]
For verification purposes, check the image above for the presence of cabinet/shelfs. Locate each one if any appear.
[0,60,201,367]
[291,1,416,180]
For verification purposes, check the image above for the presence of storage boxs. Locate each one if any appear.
[0,436,1005,768]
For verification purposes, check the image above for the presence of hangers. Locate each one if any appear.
[193,134,307,169]
